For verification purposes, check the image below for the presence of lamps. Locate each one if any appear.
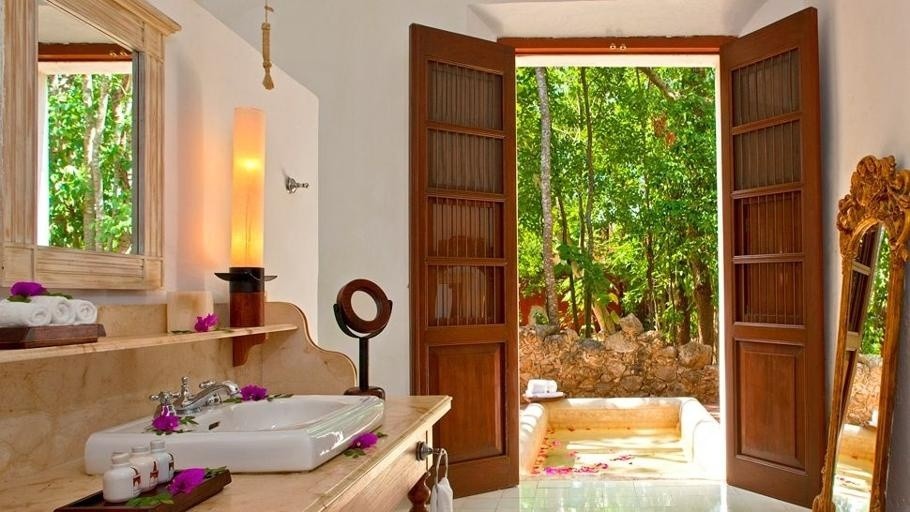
[215,104,280,366]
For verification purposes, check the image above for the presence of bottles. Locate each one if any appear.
[98,436,178,506]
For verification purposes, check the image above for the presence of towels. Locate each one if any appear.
[526,378,564,398]
[1,293,99,328]
[430,476,455,512]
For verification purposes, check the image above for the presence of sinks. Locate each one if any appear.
[86,395,385,471]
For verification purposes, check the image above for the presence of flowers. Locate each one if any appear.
[148,405,198,435]
[344,428,387,461]
[223,381,294,406]
[127,467,232,511]
[5,280,75,304]
[192,310,223,335]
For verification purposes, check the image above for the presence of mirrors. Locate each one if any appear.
[2,0,179,290]
[813,153,910,510]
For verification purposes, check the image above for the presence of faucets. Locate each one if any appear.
[174,377,242,413]
